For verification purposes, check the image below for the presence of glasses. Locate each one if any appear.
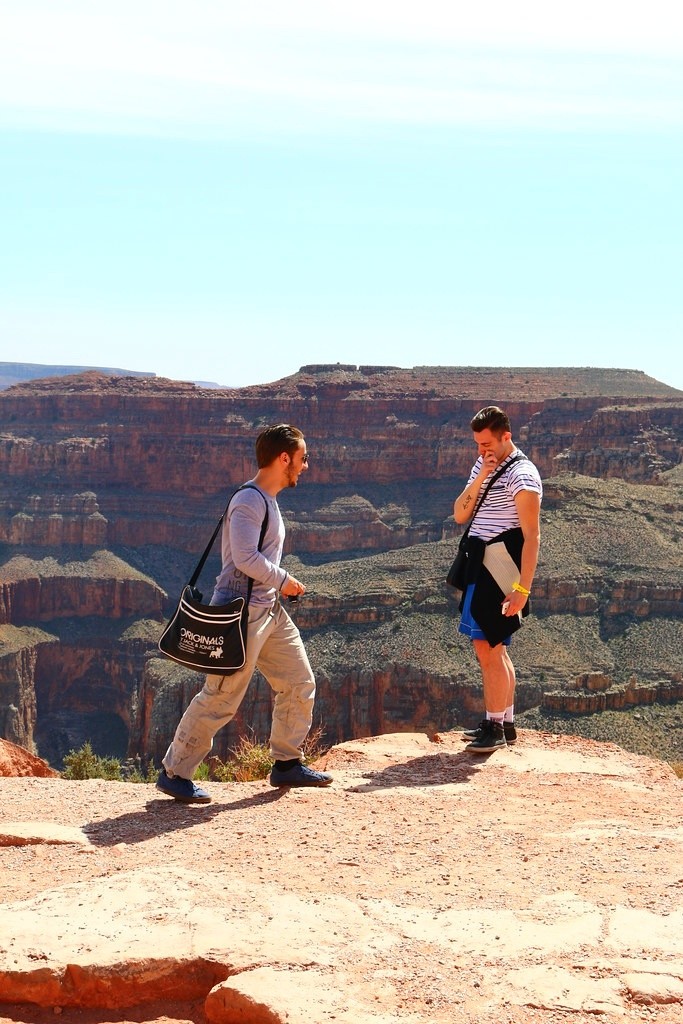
[286,450,309,464]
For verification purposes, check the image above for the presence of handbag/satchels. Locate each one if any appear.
[447,537,468,593]
[157,585,248,676]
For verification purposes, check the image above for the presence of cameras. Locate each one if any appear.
[288,595,298,604]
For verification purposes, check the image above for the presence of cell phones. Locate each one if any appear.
[502,601,510,615]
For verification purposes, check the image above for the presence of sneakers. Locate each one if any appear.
[462,719,517,744]
[465,718,507,752]
[155,769,211,803]
[269,760,334,787]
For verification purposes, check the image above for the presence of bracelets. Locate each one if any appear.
[511,582,531,597]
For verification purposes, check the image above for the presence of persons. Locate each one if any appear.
[156,424,334,803]
[454,406,543,753]
